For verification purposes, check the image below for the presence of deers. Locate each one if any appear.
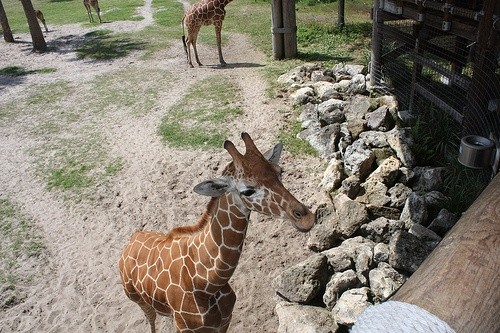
[181,0,234,68]
[82,0,102,23]
[33,10,48,32]
[119,132,315,332]
